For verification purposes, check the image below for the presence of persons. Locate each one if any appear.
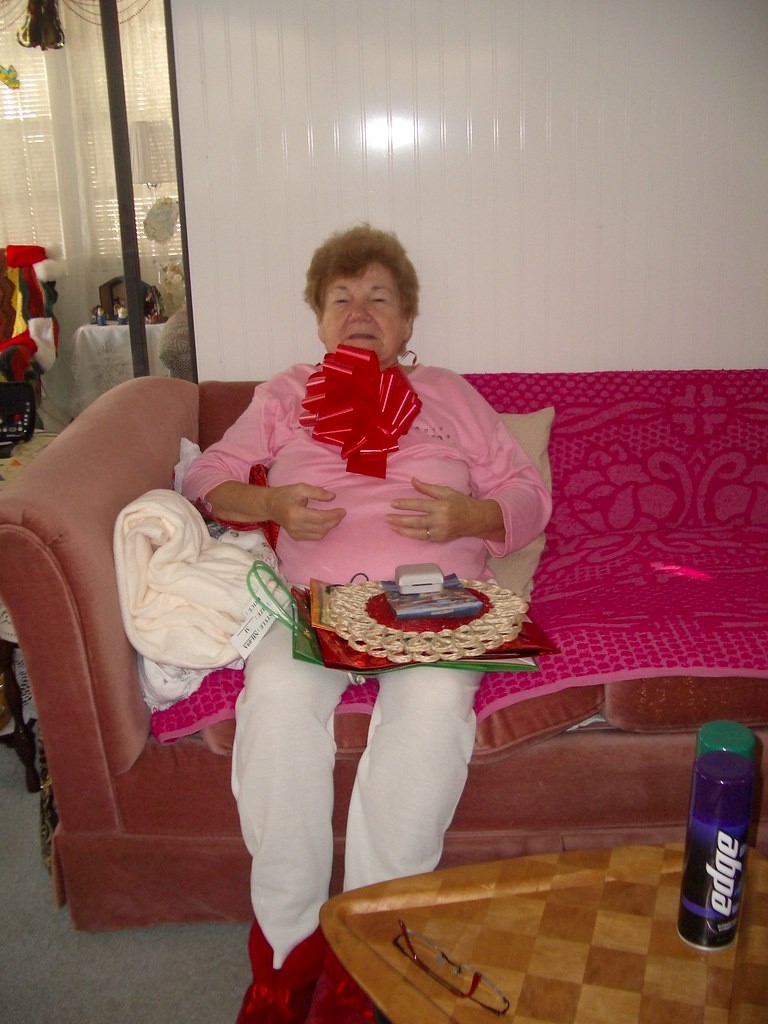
[178,226,551,1023]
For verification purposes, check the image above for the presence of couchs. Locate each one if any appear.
[0,367,768,932]
[159,307,196,384]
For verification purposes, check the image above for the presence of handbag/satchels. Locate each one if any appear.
[247,560,555,676]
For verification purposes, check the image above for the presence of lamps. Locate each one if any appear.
[131,120,180,301]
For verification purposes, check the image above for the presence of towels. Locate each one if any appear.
[112,438,291,707]
[147,368,768,745]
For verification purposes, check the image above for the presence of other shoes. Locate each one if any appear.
[305,948,377,1024]
[235,921,326,1024]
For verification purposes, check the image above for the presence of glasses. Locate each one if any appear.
[393,920,509,1017]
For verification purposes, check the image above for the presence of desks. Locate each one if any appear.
[40,319,168,424]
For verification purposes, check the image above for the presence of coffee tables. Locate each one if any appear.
[234,842,768,1024]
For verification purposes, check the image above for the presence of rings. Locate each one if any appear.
[426,527,430,539]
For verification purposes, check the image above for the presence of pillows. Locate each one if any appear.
[482,405,557,603]
